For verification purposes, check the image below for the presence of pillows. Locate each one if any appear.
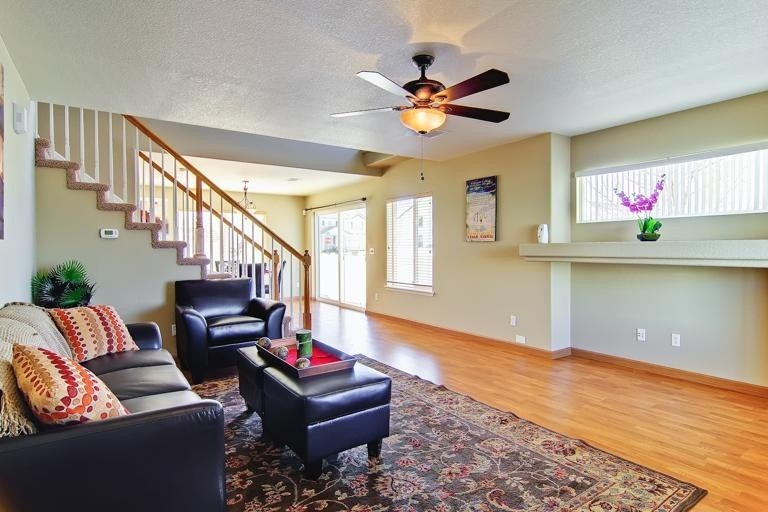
[48,305,140,363]
[12,343,131,431]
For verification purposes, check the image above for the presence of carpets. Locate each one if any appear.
[191,353,707,512]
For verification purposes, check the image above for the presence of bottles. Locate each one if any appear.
[296,330,312,359]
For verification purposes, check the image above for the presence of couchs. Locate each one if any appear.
[0,302,227,512]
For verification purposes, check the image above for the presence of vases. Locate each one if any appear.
[636,233,661,241]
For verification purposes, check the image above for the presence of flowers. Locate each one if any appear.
[611,175,666,233]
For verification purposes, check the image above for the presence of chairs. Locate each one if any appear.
[175,278,286,384]
[240,263,269,297]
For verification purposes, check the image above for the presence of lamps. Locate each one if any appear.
[234,180,257,221]
[329,53,510,124]
[400,107,447,137]
